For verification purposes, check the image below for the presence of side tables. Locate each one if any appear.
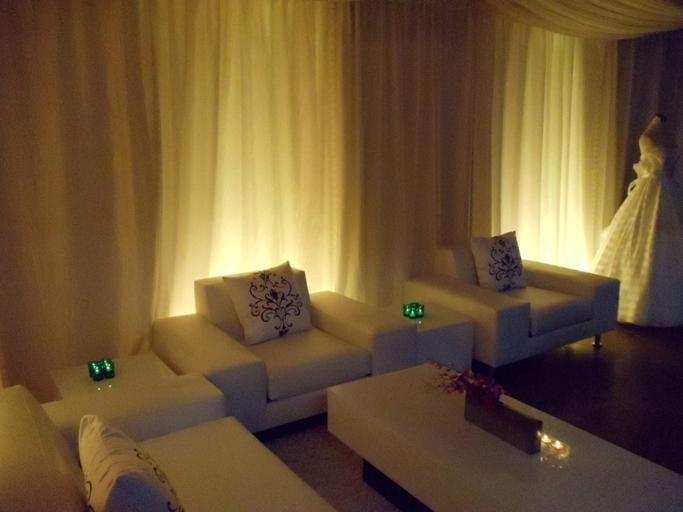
[381,299,475,372]
[52,350,176,399]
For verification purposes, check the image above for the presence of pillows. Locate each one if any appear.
[78,414,185,511]
[470,231,528,291]
[222,262,312,346]
[447,237,478,284]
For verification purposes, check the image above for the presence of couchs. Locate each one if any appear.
[0,373,337,512]
[404,246,620,370]
[150,281,417,434]
